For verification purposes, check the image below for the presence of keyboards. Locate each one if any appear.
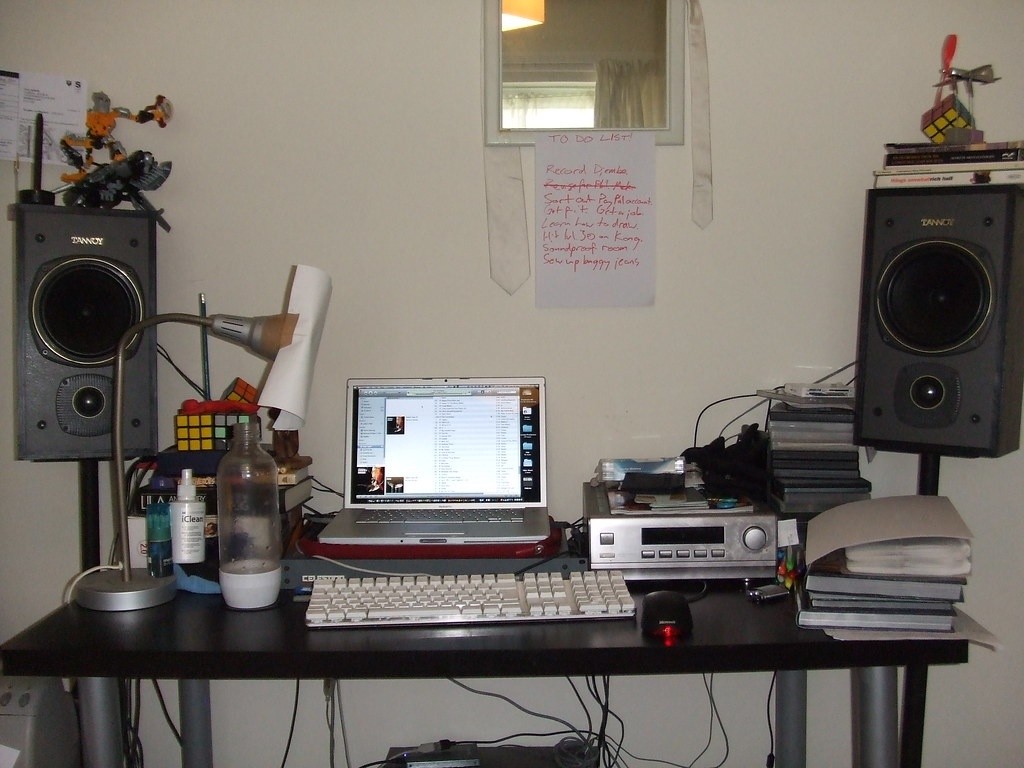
[306,570,635,628]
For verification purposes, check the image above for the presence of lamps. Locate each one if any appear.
[75,313,300,611]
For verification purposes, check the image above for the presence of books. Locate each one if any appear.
[795,495,974,634]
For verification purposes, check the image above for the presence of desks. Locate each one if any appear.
[0,577,969,768]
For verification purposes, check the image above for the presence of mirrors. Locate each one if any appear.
[481,0,685,146]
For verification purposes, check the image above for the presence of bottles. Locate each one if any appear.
[217,423,282,609]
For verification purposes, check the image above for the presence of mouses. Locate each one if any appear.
[640,590,699,644]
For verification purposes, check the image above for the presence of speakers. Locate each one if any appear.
[855,184,1024,459]
[15,203,158,461]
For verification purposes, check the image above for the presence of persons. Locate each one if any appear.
[367,467,384,494]
[392,417,403,433]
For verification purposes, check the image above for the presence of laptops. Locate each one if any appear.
[318,376,551,546]
